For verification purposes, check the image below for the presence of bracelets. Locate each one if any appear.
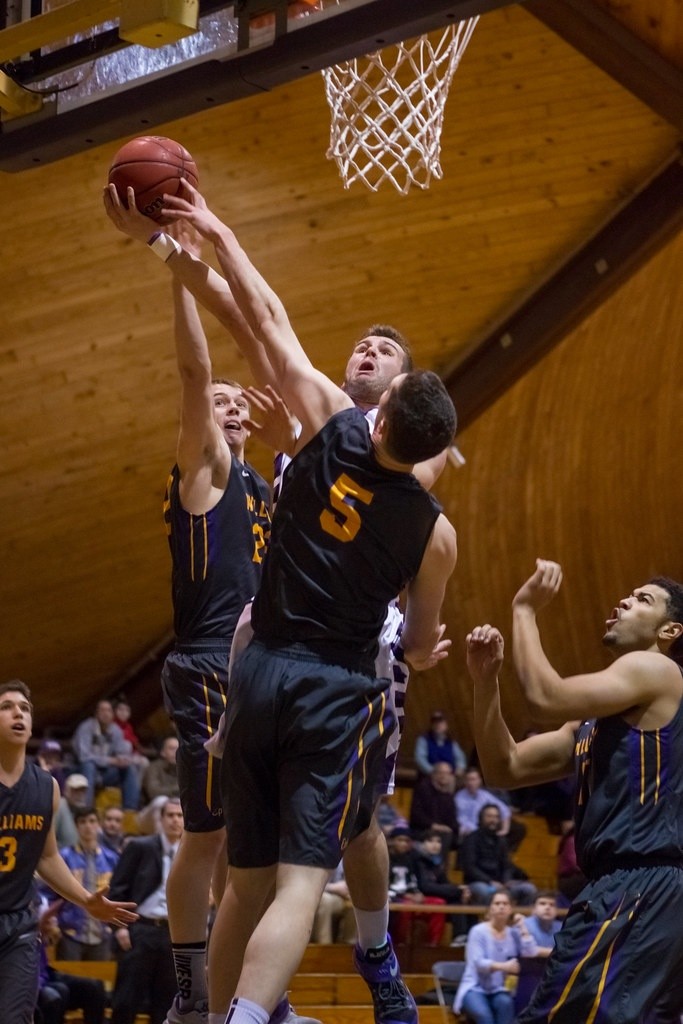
[147,229,181,262]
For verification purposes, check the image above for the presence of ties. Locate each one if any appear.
[168,849,175,872]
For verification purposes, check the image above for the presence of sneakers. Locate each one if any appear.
[267,997,322,1024]
[351,933,419,1024]
[162,993,209,1024]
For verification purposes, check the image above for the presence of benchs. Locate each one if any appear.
[46,784,570,1023]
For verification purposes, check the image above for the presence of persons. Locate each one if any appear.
[0,558,683,1024]
[101,171,467,1024]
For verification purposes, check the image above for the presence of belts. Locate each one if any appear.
[137,917,168,928]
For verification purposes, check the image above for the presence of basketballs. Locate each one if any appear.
[107,135,200,227]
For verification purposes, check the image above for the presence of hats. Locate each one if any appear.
[430,710,444,720]
[389,828,416,840]
[66,774,89,788]
[39,739,63,752]
